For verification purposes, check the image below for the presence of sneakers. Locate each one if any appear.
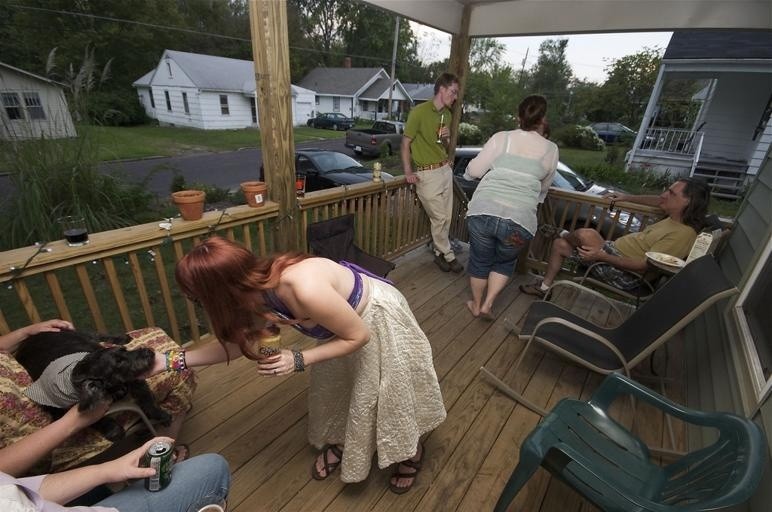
[538,223,567,239]
[434,252,451,272]
[449,258,464,274]
[519,279,552,300]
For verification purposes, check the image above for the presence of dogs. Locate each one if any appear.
[13,325,173,444]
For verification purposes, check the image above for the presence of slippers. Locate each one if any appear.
[170,444,190,464]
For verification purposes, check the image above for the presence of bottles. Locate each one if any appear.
[374,161,382,182]
[435,114,445,143]
[259,323,281,371]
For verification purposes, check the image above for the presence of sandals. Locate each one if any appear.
[388,444,424,494]
[312,445,343,481]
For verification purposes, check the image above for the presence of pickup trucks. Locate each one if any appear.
[346,120,407,157]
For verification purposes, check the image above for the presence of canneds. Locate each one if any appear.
[144,439,173,492]
[256,326,281,359]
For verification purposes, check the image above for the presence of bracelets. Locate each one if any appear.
[164,349,188,373]
[289,349,306,372]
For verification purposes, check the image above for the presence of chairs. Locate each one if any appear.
[306,212,396,282]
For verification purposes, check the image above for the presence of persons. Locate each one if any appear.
[400,73,465,274]
[462,95,559,318]
[133,234,449,495]
[0,436,231,511]
[537,116,551,139]
[518,176,711,300]
[1,317,200,479]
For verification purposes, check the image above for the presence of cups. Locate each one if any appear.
[193,493,228,512]
[295,174,306,199]
[57,217,89,244]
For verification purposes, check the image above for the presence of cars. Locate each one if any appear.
[453,147,643,235]
[587,122,658,149]
[644,128,669,150]
[306,112,355,132]
[260,148,397,211]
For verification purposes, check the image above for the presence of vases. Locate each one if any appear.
[240,179,268,208]
[171,190,207,221]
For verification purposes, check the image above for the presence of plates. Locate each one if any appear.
[645,249,685,268]
